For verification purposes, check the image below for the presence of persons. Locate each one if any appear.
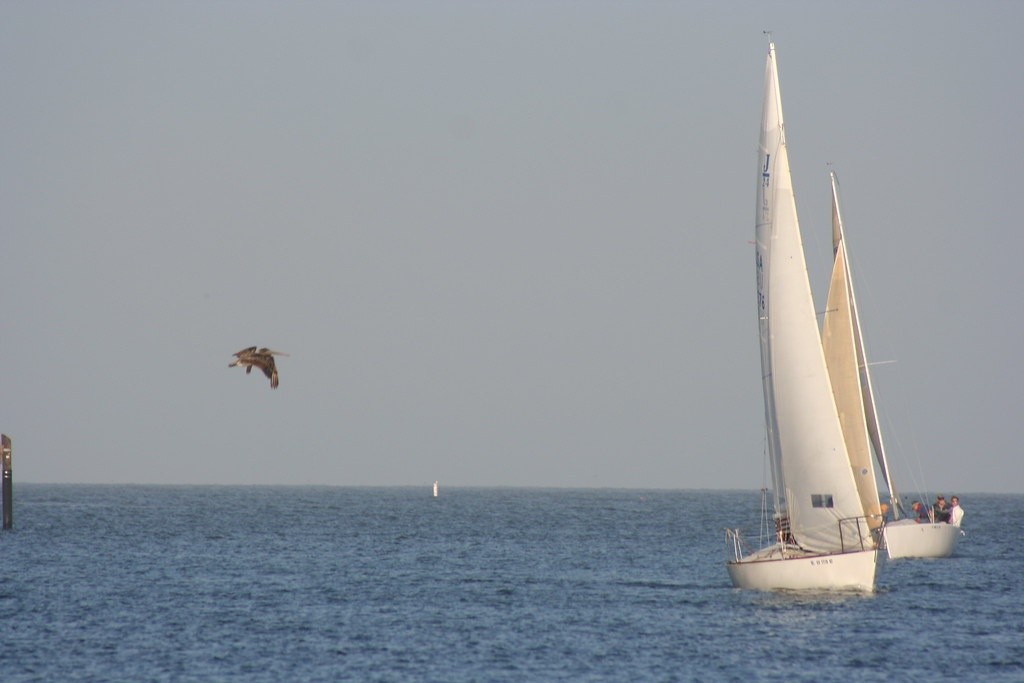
[934,494,950,523]
[948,497,965,528]
[911,501,932,523]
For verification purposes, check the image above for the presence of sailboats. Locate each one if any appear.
[820,171,967,561]
[725,30,882,603]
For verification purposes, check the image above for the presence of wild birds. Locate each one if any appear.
[228,346,291,389]
